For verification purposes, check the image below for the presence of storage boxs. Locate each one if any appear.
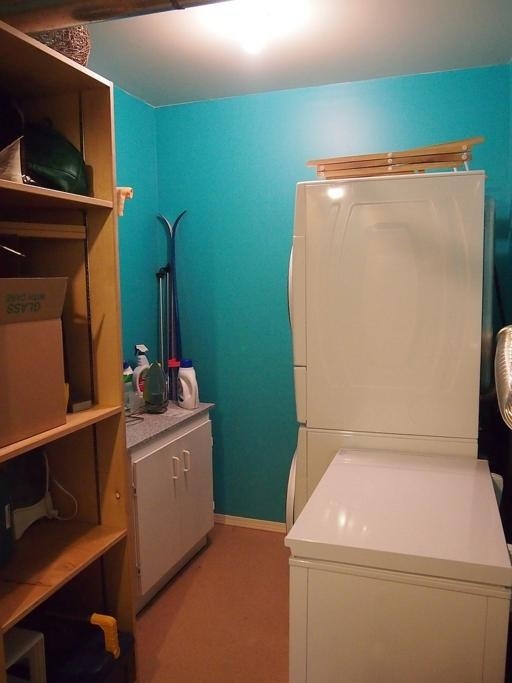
[0,275,69,452]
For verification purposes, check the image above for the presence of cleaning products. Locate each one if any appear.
[130,344,151,409]
[123,361,133,396]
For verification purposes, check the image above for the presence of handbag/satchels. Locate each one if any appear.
[20,118,88,196]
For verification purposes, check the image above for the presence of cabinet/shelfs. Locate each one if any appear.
[132,420,215,617]
[0,21,143,683]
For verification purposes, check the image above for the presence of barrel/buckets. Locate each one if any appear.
[177,360,200,409]
